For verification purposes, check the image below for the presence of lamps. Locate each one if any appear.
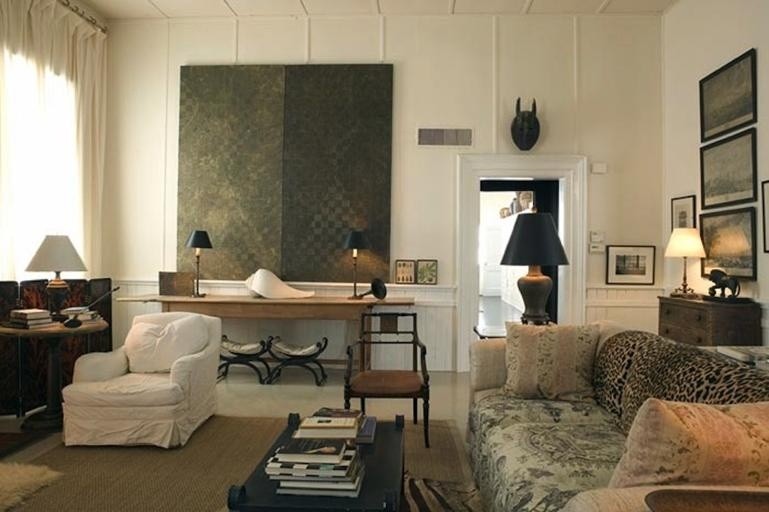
[664,228,707,299]
[24,235,88,321]
[184,230,212,298]
[501,213,570,325]
[343,229,386,300]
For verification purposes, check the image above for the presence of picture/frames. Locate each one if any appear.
[699,127,757,210]
[699,48,757,143]
[417,259,437,284]
[671,194,696,233]
[699,206,757,281]
[395,260,415,284]
[761,180,769,252]
[606,245,656,284]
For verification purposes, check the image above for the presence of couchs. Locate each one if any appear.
[61,311,223,449]
[466,320,769,512]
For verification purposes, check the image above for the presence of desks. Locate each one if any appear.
[117,293,415,371]
[0,318,108,433]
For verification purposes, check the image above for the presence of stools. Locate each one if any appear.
[215,335,270,384]
[265,336,329,386]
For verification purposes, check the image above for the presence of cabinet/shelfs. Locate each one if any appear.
[657,294,761,347]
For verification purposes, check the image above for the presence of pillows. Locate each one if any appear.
[609,397,769,487]
[120,315,209,373]
[496,321,601,402]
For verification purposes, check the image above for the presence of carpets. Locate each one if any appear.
[0,414,483,512]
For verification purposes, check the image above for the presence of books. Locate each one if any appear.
[11,308,59,328]
[62,305,103,323]
[293,408,377,443]
[265,437,365,499]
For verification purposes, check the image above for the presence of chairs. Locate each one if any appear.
[345,313,431,448]
